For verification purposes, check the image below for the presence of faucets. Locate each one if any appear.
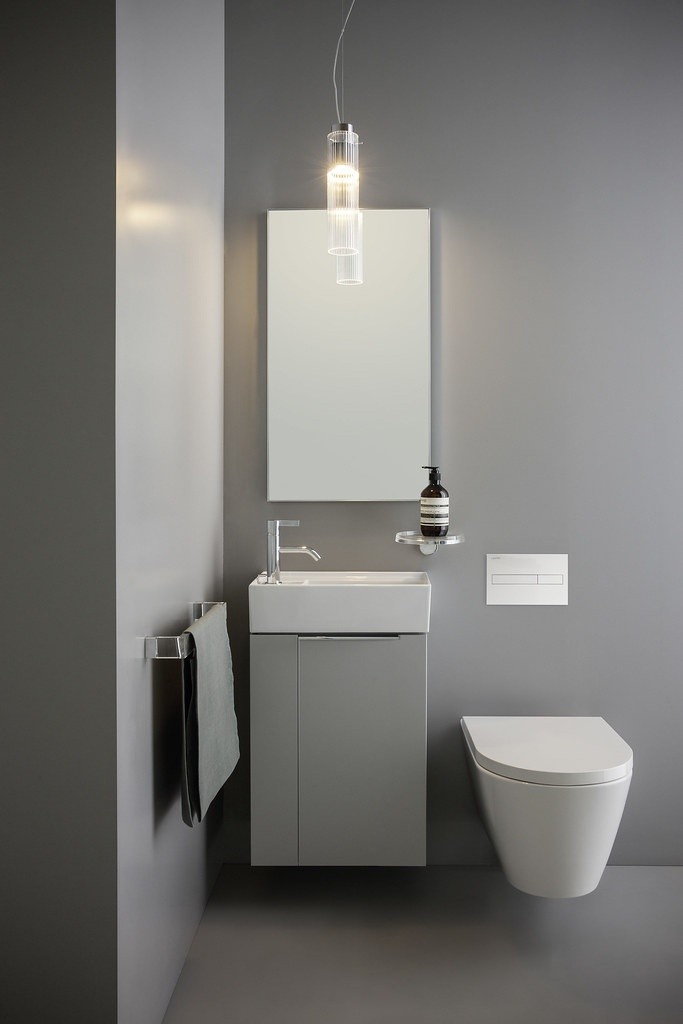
[266,519,323,584]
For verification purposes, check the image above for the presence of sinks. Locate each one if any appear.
[248,570,431,634]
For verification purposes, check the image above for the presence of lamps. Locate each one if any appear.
[327,0,366,286]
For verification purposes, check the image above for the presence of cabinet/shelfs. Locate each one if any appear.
[252,635,429,868]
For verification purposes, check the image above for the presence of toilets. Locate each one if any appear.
[460,715,634,899]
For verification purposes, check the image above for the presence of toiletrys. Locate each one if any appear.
[421,465,450,537]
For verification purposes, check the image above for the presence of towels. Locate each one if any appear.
[181,604,241,828]
